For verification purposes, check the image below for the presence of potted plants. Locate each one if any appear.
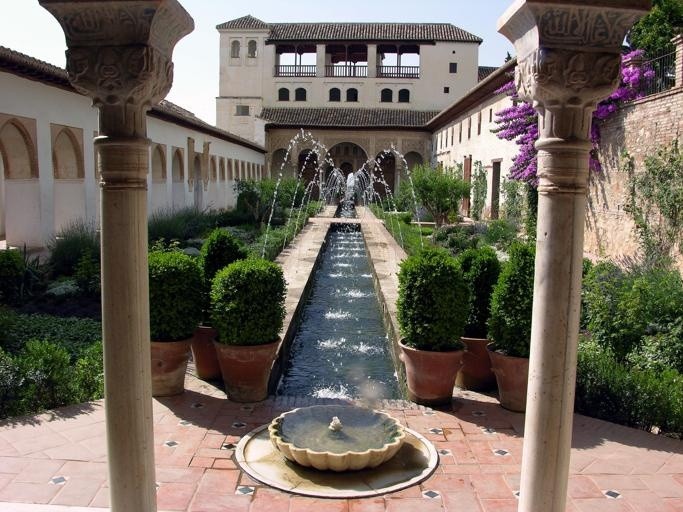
[148,228,288,402]
[394,240,535,414]
